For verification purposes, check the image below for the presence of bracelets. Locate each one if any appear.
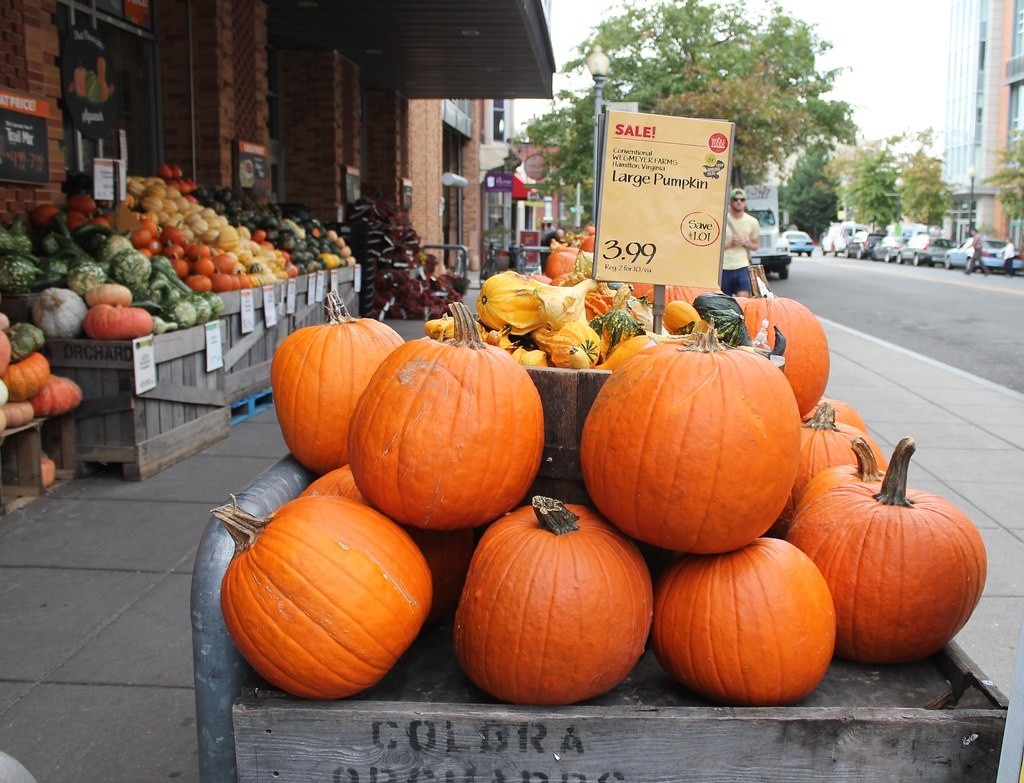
[743,242,749,247]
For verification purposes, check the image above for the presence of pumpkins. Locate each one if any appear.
[209,225,987,707]
[0,164,355,433]
[41,457,56,487]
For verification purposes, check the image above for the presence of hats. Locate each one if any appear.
[730,188,746,198]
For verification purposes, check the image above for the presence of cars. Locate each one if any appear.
[945,237,1024,273]
[782,231,814,257]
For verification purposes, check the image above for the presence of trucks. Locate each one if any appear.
[744,184,790,279]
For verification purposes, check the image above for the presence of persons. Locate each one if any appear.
[965,229,990,276]
[1002,238,1015,277]
[721,188,760,298]
[540,222,564,274]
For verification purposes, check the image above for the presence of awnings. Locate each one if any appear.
[512,173,539,200]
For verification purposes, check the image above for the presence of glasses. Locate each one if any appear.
[732,198,745,202]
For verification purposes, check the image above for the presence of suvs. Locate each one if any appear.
[845,230,953,266]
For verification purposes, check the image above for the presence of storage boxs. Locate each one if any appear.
[0,408,75,514]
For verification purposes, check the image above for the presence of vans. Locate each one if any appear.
[822,224,869,257]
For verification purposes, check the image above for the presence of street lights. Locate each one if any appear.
[967,167,978,237]
[585,43,609,227]
